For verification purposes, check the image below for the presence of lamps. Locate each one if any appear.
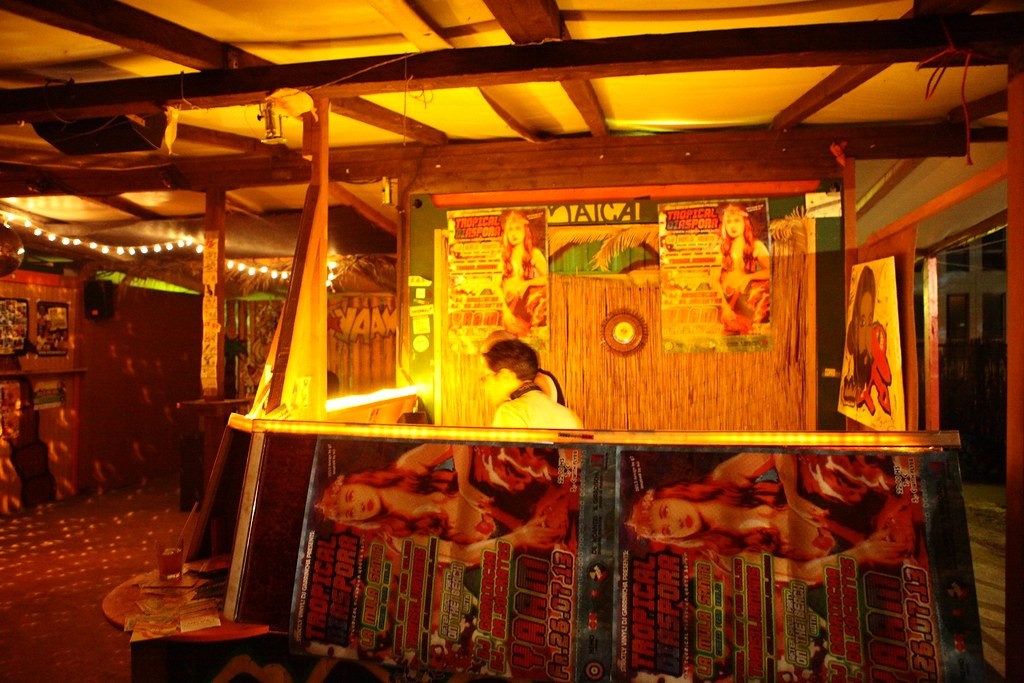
[257,102,290,147]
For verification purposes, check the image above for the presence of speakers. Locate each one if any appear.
[84,279,114,320]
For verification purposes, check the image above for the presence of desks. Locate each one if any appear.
[98,550,298,671]
[173,382,249,500]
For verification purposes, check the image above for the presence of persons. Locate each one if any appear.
[629,452,925,582]
[319,444,578,566]
[708,205,769,335]
[494,211,547,335]
[484,339,582,429]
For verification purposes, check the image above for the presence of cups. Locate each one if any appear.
[156,535,184,583]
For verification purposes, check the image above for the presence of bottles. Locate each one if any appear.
[24,346,38,370]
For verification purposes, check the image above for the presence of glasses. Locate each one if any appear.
[481,368,502,384]
